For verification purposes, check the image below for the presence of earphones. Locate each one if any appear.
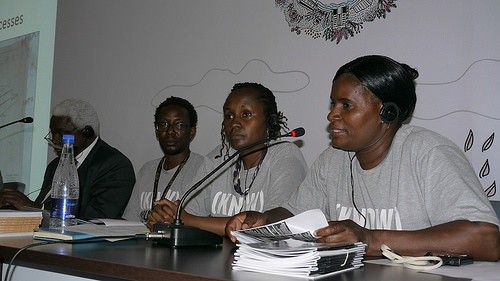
[82,126,95,138]
[379,103,399,123]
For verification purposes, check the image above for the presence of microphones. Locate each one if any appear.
[149,127,306,246]
[0,117,33,130]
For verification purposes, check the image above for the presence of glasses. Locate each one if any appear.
[43,128,77,151]
[232,155,267,195]
[153,120,191,133]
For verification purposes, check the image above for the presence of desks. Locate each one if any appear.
[0,220,500,281]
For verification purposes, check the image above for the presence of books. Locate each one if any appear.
[0,210,150,243]
[232,208,368,279]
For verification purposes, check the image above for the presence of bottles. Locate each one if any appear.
[47,135,80,231]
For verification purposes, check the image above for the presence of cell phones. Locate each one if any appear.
[438,254,473,266]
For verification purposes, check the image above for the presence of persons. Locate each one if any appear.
[147,81,308,237]
[0,98,135,227]
[225,54,500,262]
[121,96,203,222]
